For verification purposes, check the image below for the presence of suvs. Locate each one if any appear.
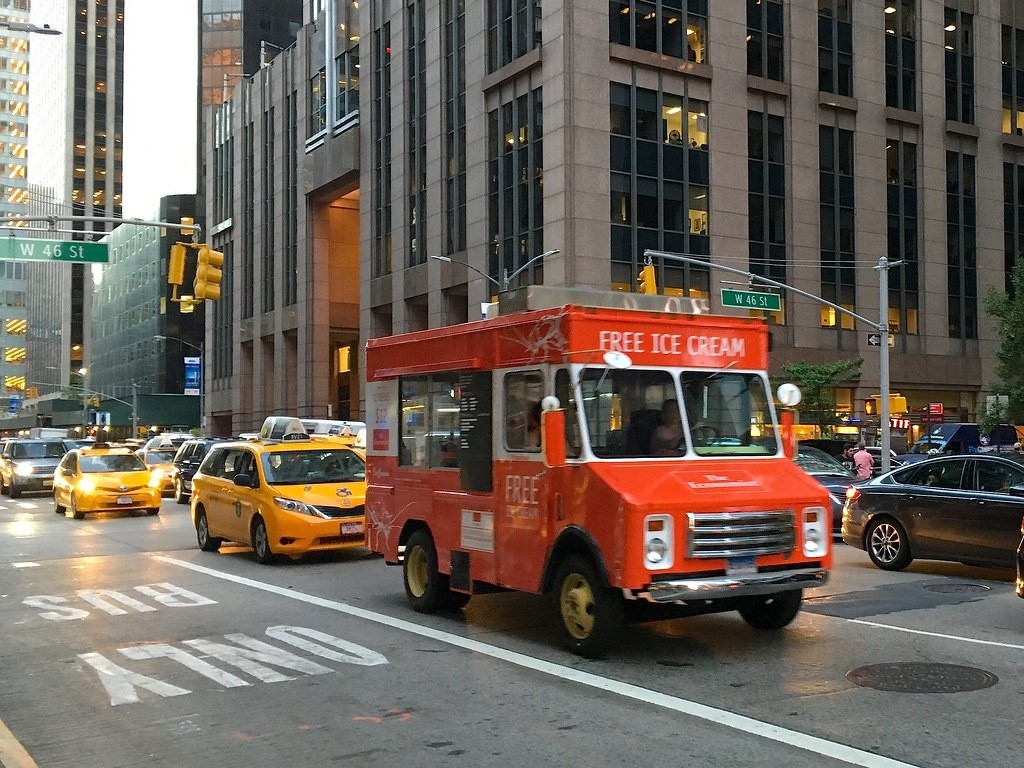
[172,436,256,504]
[0,439,69,498]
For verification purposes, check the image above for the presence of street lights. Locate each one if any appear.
[430,249,562,291]
[47,367,88,436]
[155,336,203,435]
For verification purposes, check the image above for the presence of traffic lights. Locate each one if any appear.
[26,388,31,398]
[137,427,147,434]
[638,266,657,295]
[93,398,99,407]
[196,246,224,302]
[32,387,38,398]
[864,398,881,415]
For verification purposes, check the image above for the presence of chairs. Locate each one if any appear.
[626,408,666,455]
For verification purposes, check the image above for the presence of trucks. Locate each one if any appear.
[30,429,69,438]
[364,303,833,656]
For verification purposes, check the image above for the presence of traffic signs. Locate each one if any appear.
[867,333,895,348]
[0,236,109,264]
[721,288,781,311]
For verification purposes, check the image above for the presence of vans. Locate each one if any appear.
[892,424,1020,465]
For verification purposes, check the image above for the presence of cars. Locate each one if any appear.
[190,416,382,565]
[768,444,856,533]
[135,438,179,495]
[853,447,904,480]
[52,444,162,519]
[839,454,1024,599]
[62,437,148,466]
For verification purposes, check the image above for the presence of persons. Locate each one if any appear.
[835,443,858,475]
[649,399,684,457]
[853,443,874,480]
